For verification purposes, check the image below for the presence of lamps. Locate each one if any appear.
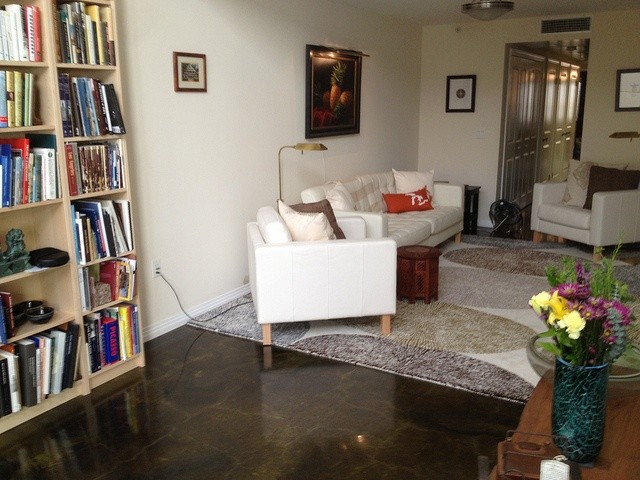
[461,0,514,23]
[274,142,327,199]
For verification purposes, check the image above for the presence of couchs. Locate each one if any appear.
[244,219,400,345]
[301,170,466,248]
[528,163,640,258]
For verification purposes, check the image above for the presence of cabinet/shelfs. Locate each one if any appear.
[502,48,546,208]
[0,2,148,434]
[538,57,581,182]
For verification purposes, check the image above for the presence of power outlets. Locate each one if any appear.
[151,266,162,278]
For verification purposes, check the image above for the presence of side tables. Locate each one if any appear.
[437,179,482,232]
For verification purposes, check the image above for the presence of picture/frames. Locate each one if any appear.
[300,41,363,141]
[443,73,478,114]
[171,50,210,94]
[612,67,639,113]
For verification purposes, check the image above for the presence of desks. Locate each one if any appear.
[488,369,639,478]
[398,243,443,305]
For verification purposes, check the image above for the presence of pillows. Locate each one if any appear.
[581,164,638,211]
[327,181,359,211]
[380,186,434,215]
[291,198,345,239]
[391,167,436,193]
[277,200,336,240]
[561,156,627,207]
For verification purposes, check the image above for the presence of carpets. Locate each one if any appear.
[184,232,639,404]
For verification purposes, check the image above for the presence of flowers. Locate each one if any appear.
[525,228,640,369]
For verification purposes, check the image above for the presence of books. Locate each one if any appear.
[3,136,62,208]
[71,201,135,265]
[55,0,116,65]
[66,142,127,193]
[58,71,127,138]
[80,258,136,310]
[0,291,17,347]
[1,71,39,129]
[81,304,141,374]
[0,323,80,414]
[0,4,43,63]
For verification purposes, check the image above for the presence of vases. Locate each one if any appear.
[547,355,612,467]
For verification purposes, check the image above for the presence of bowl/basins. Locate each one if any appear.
[17,300,44,314]
[13,307,26,326]
[26,306,54,324]
